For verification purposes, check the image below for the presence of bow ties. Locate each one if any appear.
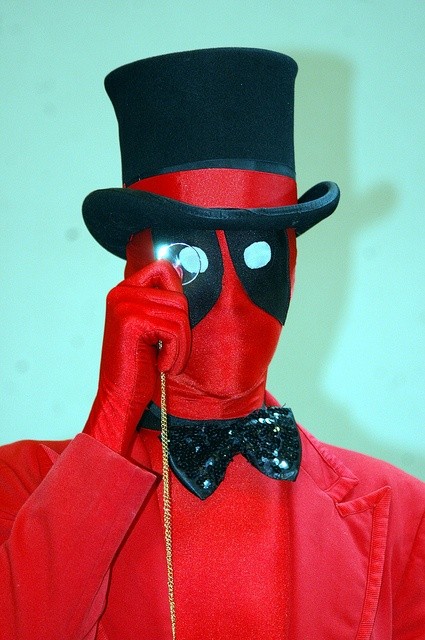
[134,402,301,500]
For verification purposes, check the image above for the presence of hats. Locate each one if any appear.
[82,46,342,261]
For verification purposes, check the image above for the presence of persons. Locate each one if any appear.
[2,47,425,640]
